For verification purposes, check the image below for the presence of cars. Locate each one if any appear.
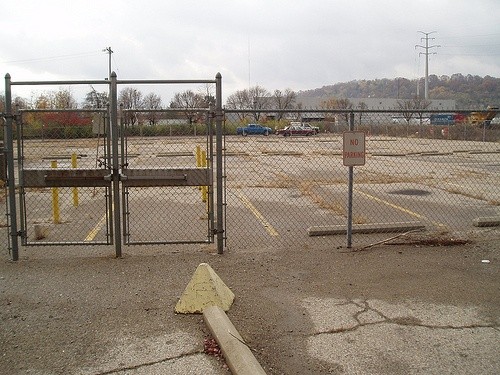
[284,121,320,135]
[276,125,316,137]
[237,123,272,136]
